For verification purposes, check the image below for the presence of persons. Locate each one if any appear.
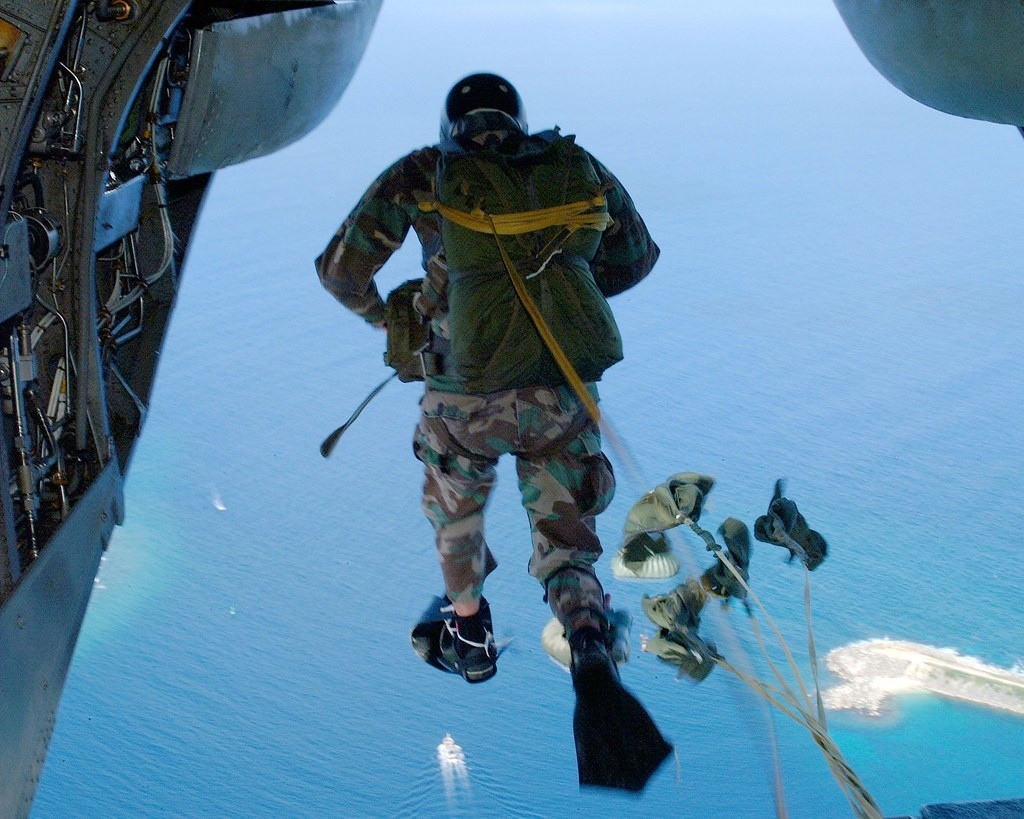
[312,72,672,793]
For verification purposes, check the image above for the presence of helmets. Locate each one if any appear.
[438,72,528,145]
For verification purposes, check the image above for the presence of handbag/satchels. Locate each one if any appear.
[382,277,428,383]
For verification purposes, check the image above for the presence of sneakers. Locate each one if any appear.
[412,622,495,680]
[570,634,624,684]
